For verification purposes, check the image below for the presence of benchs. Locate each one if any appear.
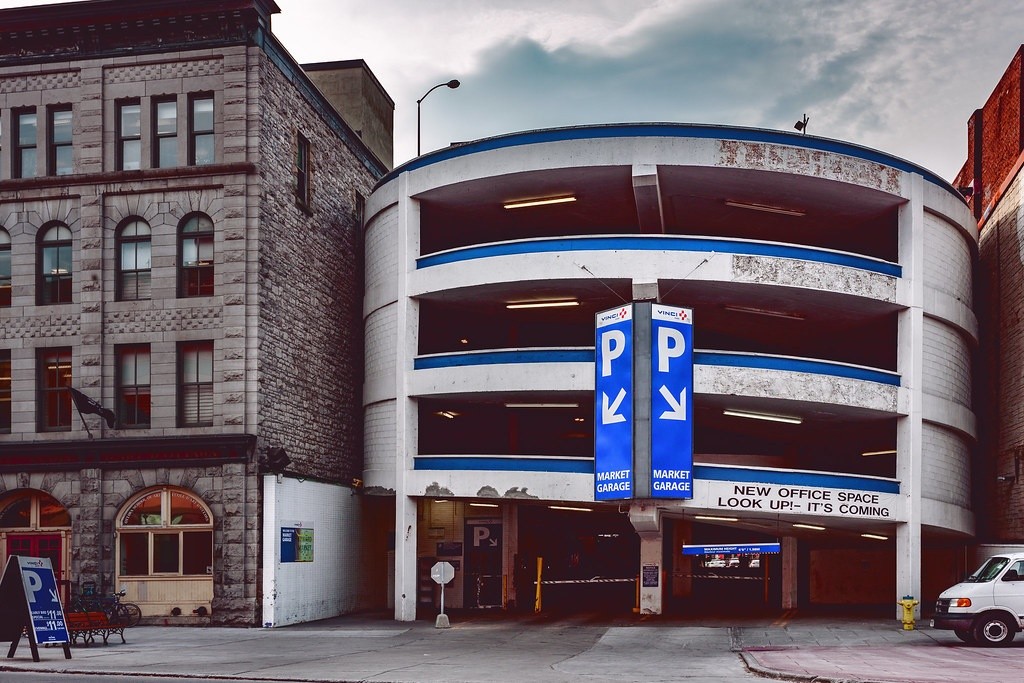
[63,612,127,647]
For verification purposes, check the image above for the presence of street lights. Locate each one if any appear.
[417,79,461,158]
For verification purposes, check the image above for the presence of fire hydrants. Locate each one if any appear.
[897,595,920,630]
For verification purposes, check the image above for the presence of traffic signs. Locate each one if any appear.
[16,558,70,645]
[594,302,636,499]
[652,306,695,499]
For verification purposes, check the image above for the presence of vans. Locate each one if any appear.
[929,553,1023,647]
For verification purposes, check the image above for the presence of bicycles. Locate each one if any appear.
[69,589,142,627]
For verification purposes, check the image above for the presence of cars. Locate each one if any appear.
[705,558,759,567]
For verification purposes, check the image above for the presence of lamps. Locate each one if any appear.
[267,446,292,470]
[997,475,1015,481]
[794,120,804,131]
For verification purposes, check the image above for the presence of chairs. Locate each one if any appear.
[1008,570,1018,580]
